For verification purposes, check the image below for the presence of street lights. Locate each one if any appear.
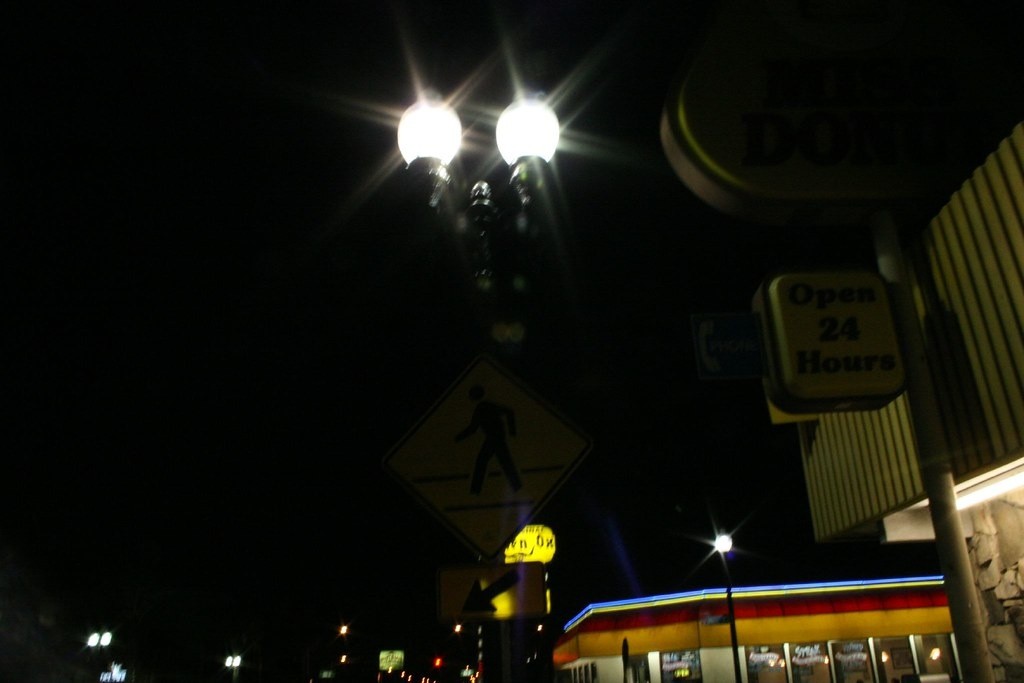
[714,534,741,683]
[391,87,565,683]
